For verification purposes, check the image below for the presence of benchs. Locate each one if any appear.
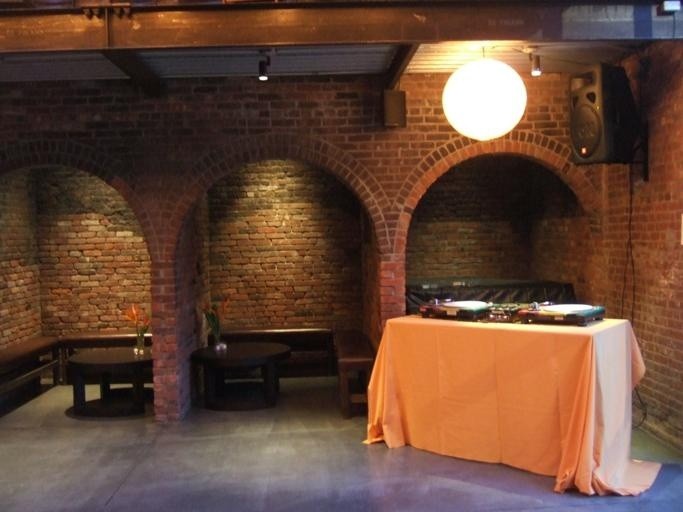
[335,330,375,419]
[0,336,59,396]
[208,328,336,376]
[59,333,152,384]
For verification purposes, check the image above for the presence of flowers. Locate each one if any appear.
[123,303,152,352]
[200,293,231,344]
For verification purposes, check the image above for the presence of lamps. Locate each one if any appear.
[531,54,542,77]
[257,46,276,81]
[442,47,527,141]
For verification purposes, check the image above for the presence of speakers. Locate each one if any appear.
[568,62,634,165]
[382,89,406,128]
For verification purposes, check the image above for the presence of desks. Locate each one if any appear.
[66,347,152,415]
[192,342,291,409]
[362,314,662,496]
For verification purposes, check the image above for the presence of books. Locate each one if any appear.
[484,301,531,324]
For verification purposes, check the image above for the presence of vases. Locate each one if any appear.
[133,326,147,356]
[214,326,228,353]
